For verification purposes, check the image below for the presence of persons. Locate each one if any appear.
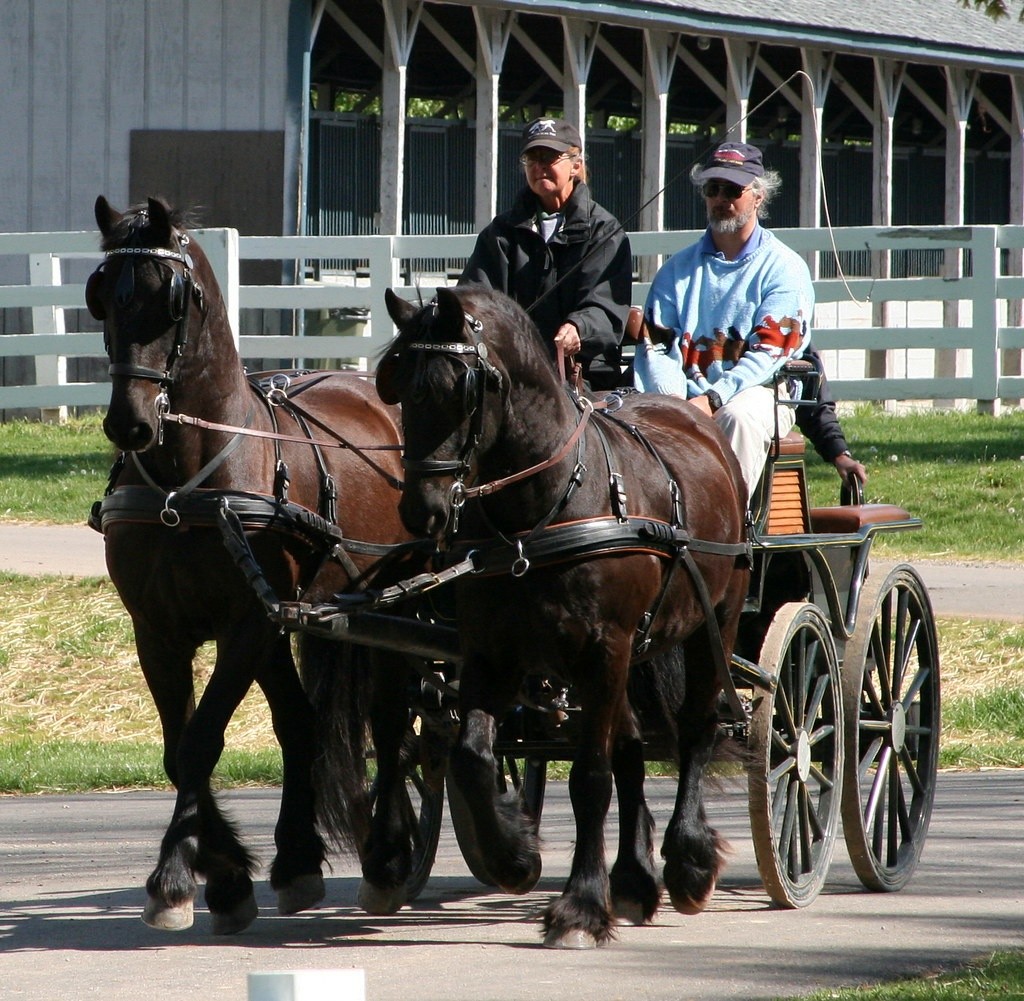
[633,141,867,507]
[457,117,631,390]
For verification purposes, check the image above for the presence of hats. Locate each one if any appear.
[519,116,582,157]
[699,142,765,188]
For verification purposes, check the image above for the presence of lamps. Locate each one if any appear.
[698,37,711,50]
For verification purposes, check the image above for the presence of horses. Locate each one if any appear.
[83,193,746,955]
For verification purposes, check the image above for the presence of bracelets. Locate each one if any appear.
[842,450,852,458]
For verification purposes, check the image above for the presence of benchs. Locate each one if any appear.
[625,307,822,539]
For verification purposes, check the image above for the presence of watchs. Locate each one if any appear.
[704,389,722,410]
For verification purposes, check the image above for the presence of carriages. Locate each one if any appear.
[82,192,943,956]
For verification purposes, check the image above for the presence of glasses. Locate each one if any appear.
[516,153,575,166]
[701,183,744,199]
